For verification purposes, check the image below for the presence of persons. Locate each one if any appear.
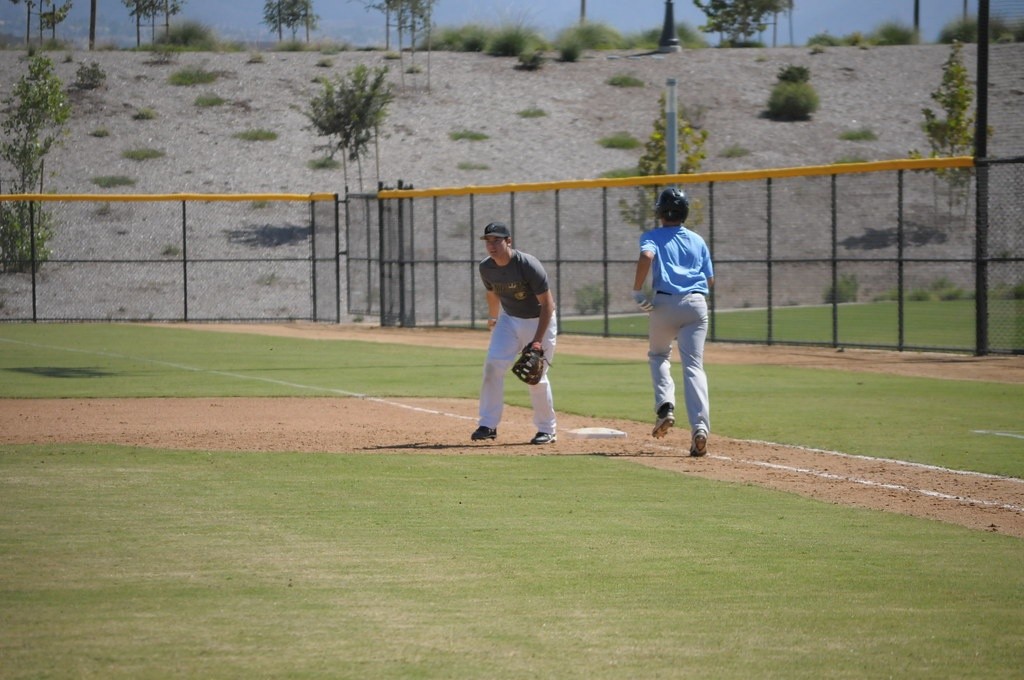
[631,187,715,457]
[471,222,557,444]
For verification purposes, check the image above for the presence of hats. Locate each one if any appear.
[480,222,510,238]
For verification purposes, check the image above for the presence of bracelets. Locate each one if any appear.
[488,317,497,321]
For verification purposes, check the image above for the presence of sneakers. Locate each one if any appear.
[471,426,496,441]
[690,430,708,455]
[531,432,555,444]
[652,403,675,439]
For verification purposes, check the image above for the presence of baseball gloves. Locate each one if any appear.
[511,341,553,386]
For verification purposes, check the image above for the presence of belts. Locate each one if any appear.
[657,291,702,295]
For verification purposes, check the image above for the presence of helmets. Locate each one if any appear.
[658,187,689,221]
